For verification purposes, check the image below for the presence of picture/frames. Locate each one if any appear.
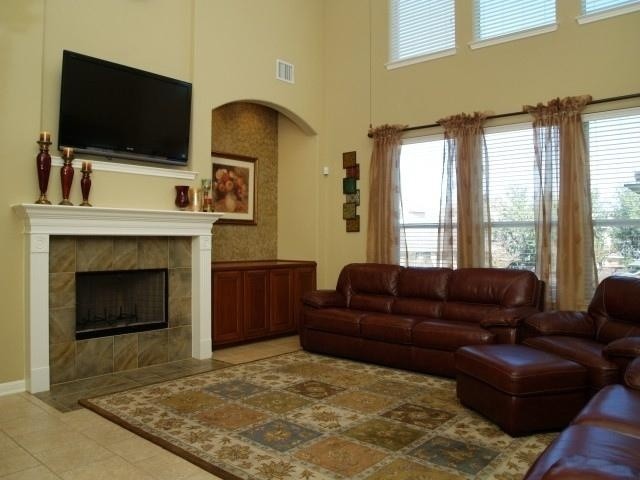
[212,150,259,226]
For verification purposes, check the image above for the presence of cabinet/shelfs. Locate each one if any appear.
[211,259,317,350]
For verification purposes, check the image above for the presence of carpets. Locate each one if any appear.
[76,351,561,479]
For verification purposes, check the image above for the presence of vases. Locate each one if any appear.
[175,185,189,210]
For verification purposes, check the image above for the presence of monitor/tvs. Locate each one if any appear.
[58,50,192,166]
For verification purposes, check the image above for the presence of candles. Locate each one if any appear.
[81,161,92,171]
[40,131,51,142]
[63,147,74,158]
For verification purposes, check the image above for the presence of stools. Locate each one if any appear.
[456,341,588,438]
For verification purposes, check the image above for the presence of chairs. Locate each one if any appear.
[523,273,639,387]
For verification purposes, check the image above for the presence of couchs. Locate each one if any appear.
[298,262,546,379]
[523,358,639,480]
